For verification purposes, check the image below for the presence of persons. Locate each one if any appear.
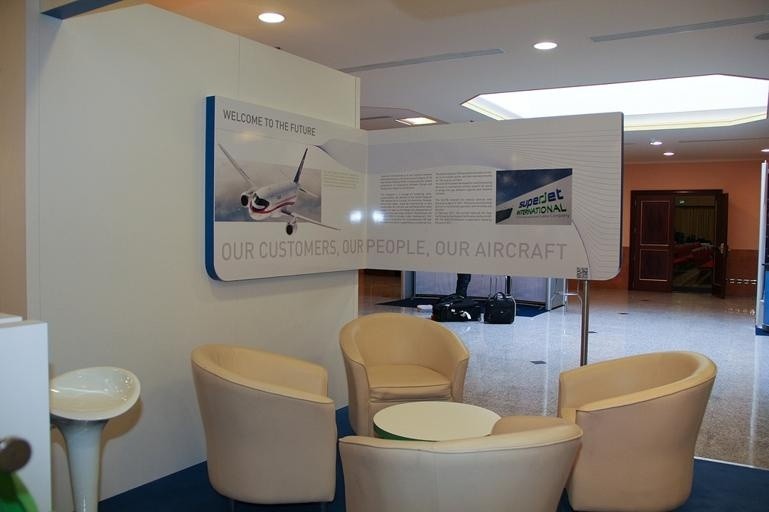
[456,274,471,297]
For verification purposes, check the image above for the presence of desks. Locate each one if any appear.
[372,401,501,442]
[0,321,52,512]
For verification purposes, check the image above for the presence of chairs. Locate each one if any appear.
[556,350,715,511]
[192,347,336,505]
[339,415,581,512]
[48,366,139,512]
[339,312,470,437]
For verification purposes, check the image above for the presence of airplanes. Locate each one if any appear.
[218,137,343,239]
